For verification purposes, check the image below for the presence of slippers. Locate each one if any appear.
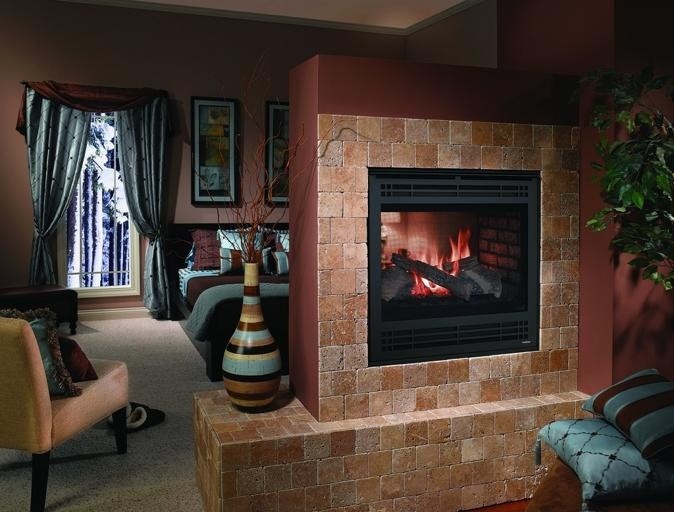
[107,401,151,426]
[125,407,166,433]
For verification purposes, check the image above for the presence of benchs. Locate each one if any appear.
[0,283,78,335]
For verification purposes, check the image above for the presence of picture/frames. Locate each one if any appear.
[189,95,240,206]
[263,100,289,207]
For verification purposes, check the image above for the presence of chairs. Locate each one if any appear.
[0,316,130,510]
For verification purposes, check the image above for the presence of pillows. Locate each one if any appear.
[534,366,673,512]
[183,223,288,277]
[0,308,97,399]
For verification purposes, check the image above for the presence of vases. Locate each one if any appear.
[221,263,283,413]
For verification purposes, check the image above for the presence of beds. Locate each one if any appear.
[164,220,288,382]
